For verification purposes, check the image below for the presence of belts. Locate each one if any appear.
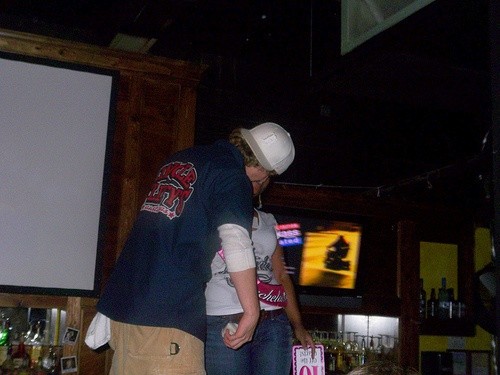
[234,308,284,324]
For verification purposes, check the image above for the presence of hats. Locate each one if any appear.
[241,122,295,175]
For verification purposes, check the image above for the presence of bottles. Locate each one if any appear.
[0,317,59,375]
[291,328,399,375]
[417,278,466,326]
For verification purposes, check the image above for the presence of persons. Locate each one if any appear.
[85,122,294,375]
[203,175,316,374]
